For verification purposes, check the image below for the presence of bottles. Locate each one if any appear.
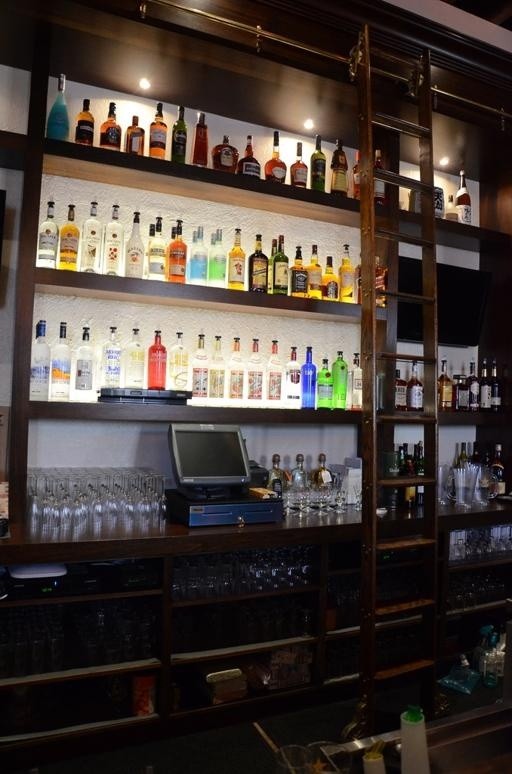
[148,216,166,281]
[479,358,491,410]
[309,453,333,491]
[46,73,70,140]
[399,446,407,476]
[211,135,239,174]
[301,346,316,410]
[208,336,227,406]
[73,327,95,403]
[245,338,266,407]
[143,223,156,278]
[321,256,339,302]
[166,332,190,391]
[338,243,356,302]
[452,373,468,411]
[456,442,469,465]
[147,331,167,389]
[437,359,453,411]
[190,227,208,286]
[405,455,416,502]
[267,453,287,491]
[272,235,289,295]
[51,322,71,401]
[186,231,197,284]
[29,319,51,401]
[189,334,208,406]
[305,245,323,299]
[78,201,101,274]
[288,454,307,491]
[330,138,348,198]
[354,252,362,304]
[237,135,261,179]
[490,359,501,411]
[382,451,400,478]
[57,204,80,271]
[316,359,333,410]
[208,233,216,255]
[100,102,122,151]
[352,151,360,199]
[467,361,479,411]
[171,105,188,164]
[491,443,506,495]
[123,115,145,156]
[123,328,145,389]
[285,347,301,409]
[406,361,424,412]
[347,353,363,409]
[374,256,387,307]
[332,351,348,412]
[417,447,425,493]
[73,98,95,146]
[267,239,278,294]
[310,133,327,192]
[454,169,472,225]
[267,340,284,408]
[35,201,59,269]
[400,706,431,774]
[208,229,226,288]
[227,229,246,290]
[102,204,125,276]
[168,220,187,284]
[289,141,308,189]
[190,111,208,167]
[395,369,407,411]
[227,337,245,407]
[264,131,287,184]
[290,245,308,298]
[101,327,122,388]
[165,227,177,282]
[248,234,269,293]
[125,212,144,279]
[149,103,168,160]
[374,149,385,206]
[469,441,481,465]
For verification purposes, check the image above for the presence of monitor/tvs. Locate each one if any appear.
[167,423,252,501]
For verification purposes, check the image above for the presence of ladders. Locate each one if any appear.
[349,21,439,700]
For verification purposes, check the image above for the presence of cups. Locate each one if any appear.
[363,752,385,774]
[284,482,349,516]
[275,746,312,774]
[352,483,365,511]
[28,466,168,521]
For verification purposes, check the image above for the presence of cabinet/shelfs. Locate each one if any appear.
[1,1,512,774]
